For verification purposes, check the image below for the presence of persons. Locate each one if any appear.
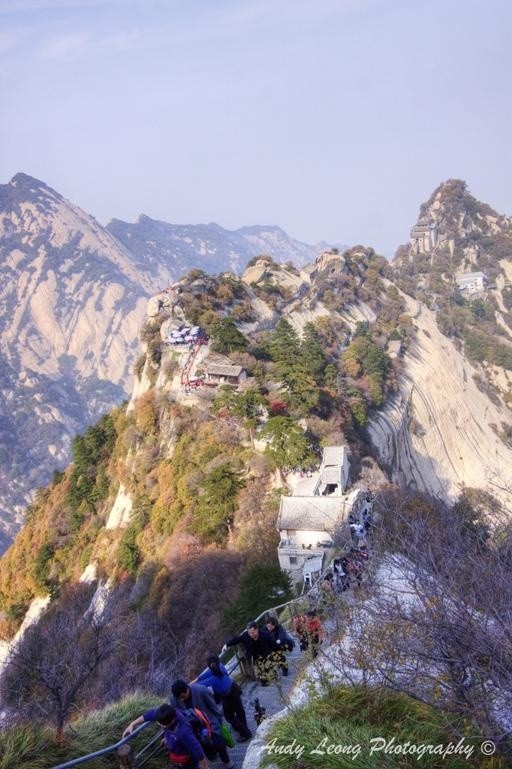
[121,487,375,769]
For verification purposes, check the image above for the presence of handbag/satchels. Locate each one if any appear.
[220,722,235,747]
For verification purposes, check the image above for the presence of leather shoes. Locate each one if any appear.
[237,733,251,742]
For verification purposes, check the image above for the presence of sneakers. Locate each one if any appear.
[261,677,268,686]
[223,757,235,767]
[282,666,289,676]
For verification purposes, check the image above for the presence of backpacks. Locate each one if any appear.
[172,706,211,749]
